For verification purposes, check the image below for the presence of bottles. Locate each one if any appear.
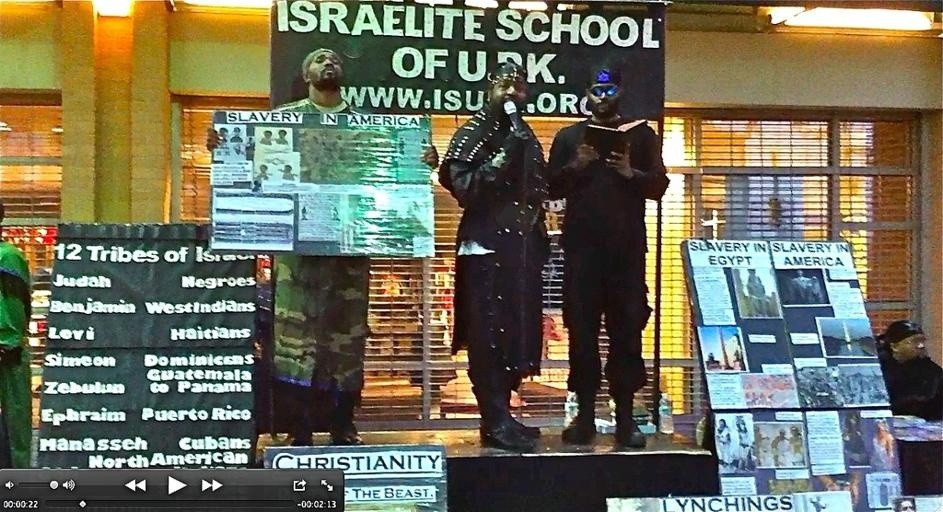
[563,390,581,425]
[659,394,674,435]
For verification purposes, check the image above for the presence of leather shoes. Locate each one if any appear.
[482,420,538,451]
[295,425,363,445]
[562,418,645,448]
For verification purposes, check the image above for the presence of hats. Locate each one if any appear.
[589,68,624,86]
[302,49,332,70]
[886,320,923,342]
[490,63,524,83]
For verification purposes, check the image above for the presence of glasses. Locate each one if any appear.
[592,87,617,96]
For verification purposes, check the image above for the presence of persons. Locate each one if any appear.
[791,270,817,304]
[547,66,671,447]
[438,62,563,454]
[219,128,293,181]
[870,418,894,473]
[207,48,439,446]
[880,320,943,423]
[895,498,915,512]
[716,418,805,471]
[0,203,33,468]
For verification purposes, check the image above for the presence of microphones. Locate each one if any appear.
[503,99,522,132]
